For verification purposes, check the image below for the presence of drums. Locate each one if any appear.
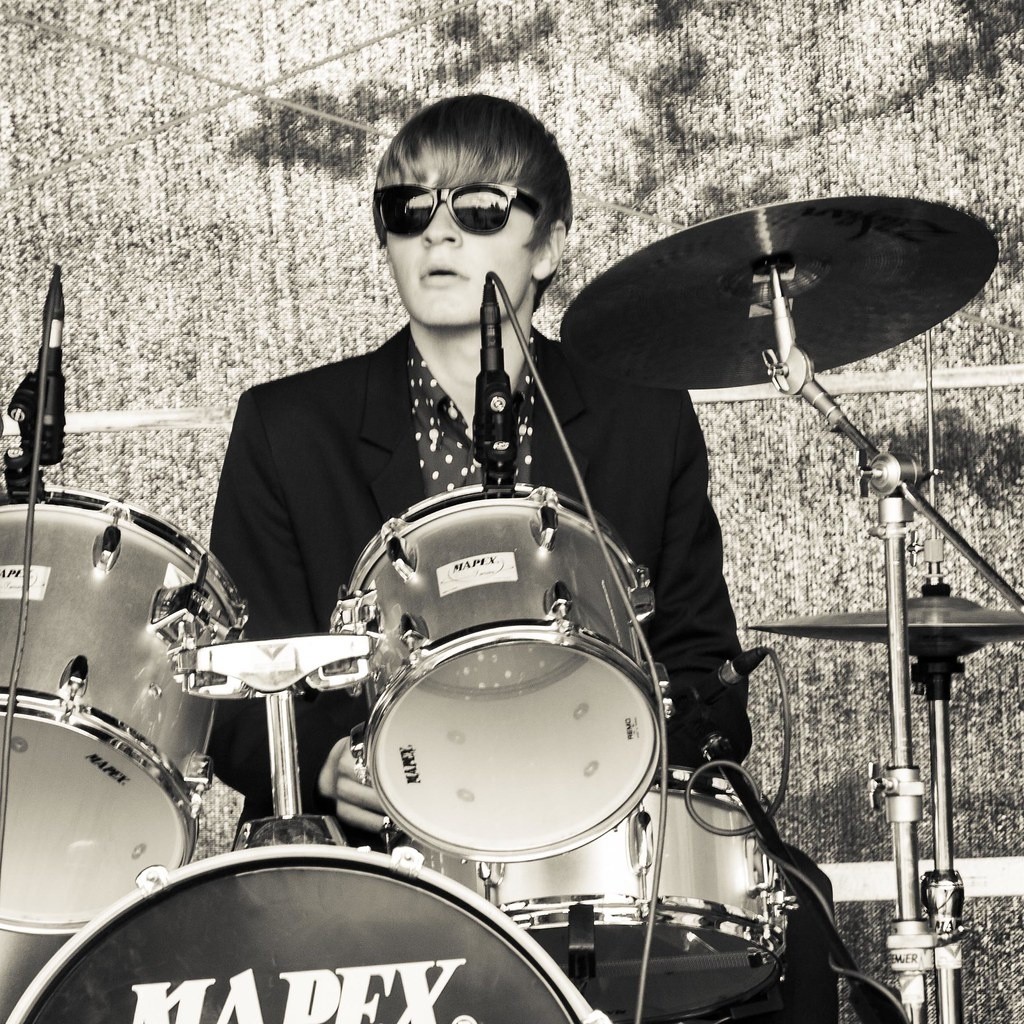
[389,757,789,1023]
[0,481,255,938]
[326,481,659,866]
[2,843,618,1024]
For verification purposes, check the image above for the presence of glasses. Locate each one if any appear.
[374,182,542,236]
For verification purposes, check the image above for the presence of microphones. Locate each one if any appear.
[472,281,521,466]
[21,280,65,465]
[668,645,769,716]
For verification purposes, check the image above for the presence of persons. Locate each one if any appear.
[203,92,754,856]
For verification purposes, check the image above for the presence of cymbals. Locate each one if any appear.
[557,194,999,393]
[747,596,1023,658]
